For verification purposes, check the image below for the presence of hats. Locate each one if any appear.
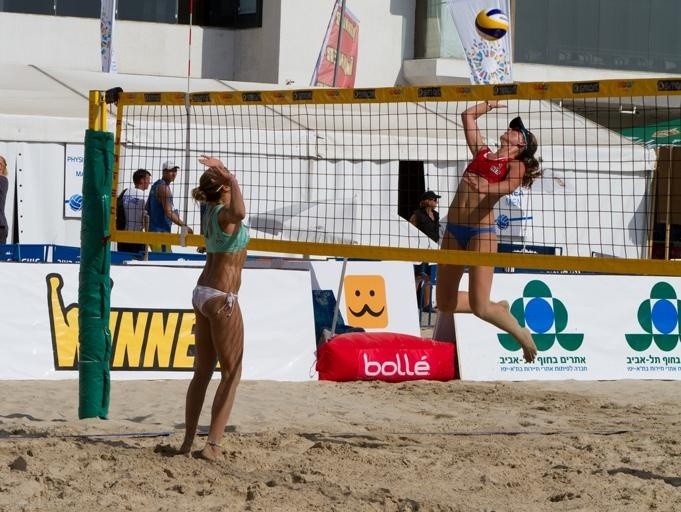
[422,191,441,199]
[510,117,532,155]
[162,161,180,170]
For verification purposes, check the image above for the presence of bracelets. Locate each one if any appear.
[485,101,492,111]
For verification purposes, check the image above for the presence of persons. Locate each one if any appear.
[436,100,544,363]
[409,191,442,312]
[0,156,8,244]
[117,169,151,253]
[145,161,193,253]
[178,155,250,460]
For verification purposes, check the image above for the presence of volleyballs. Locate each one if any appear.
[475,9,509,41]
